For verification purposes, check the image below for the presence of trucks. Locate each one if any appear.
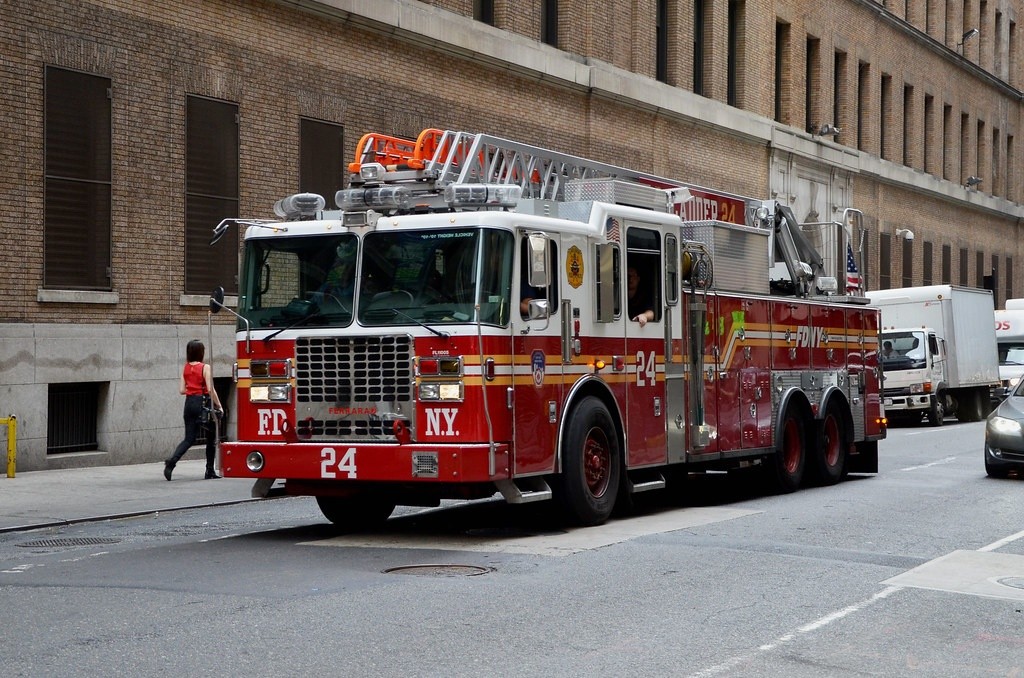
[864,284,1002,426]
[989,298,1024,403]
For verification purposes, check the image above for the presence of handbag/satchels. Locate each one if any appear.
[200,406,223,427]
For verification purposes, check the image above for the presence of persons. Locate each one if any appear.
[883,341,899,360]
[628,268,655,328]
[164,339,224,482]
[310,238,375,313]
[489,252,539,314]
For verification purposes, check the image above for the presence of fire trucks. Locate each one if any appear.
[209,128,888,528]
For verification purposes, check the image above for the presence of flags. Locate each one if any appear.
[846,242,863,291]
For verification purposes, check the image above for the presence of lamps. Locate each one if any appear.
[964,175,982,190]
[818,124,842,136]
[956,29,978,52]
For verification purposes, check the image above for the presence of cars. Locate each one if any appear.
[985,374,1024,479]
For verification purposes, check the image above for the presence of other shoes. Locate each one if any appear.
[205,465,222,479]
[163,459,176,481]
